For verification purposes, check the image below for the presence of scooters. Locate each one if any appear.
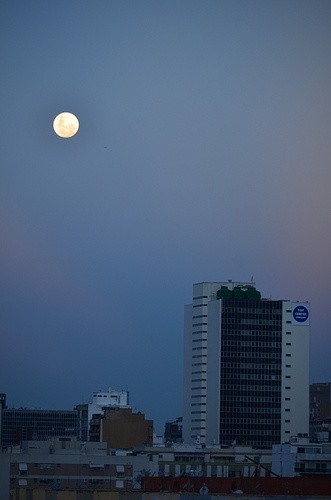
[228,489,244,497]
